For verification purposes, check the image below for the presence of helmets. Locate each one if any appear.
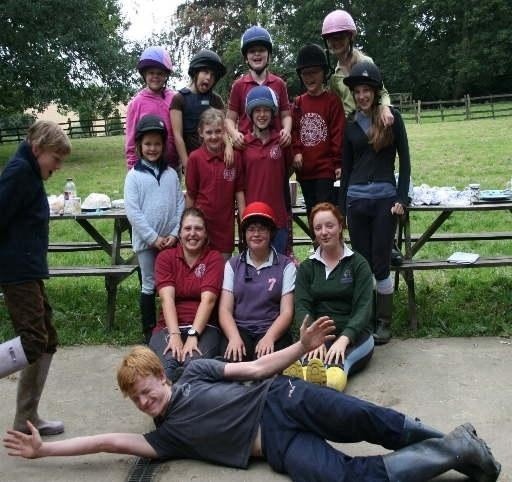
[81,193,112,210]
[240,202,276,229]
[136,10,383,140]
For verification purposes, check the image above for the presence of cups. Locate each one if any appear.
[71,197,81,214]
[289,181,298,206]
[95,198,104,213]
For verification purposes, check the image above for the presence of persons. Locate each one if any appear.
[214,200,297,363]
[281,201,375,394]
[146,207,225,384]
[1,117,78,436]
[3,311,501,482]
[123,7,410,346]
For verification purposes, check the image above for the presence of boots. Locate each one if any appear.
[0,335,65,436]
[141,291,156,346]
[380,414,501,482]
[372,290,393,344]
[282,358,348,393]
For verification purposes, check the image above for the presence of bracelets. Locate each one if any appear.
[165,331,183,344]
[187,329,200,342]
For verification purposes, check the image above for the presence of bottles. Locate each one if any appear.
[64,178,77,213]
[470,184,479,206]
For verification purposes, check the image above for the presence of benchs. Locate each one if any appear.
[46,237,132,253]
[232,232,511,242]
[389,253,512,330]
[45,263,138,329]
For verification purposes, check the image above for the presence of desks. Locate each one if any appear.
[48,187,134,333]
[292,181,511,339]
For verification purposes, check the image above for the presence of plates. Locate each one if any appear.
[477,190,510,199]
[82,206,110,211]
